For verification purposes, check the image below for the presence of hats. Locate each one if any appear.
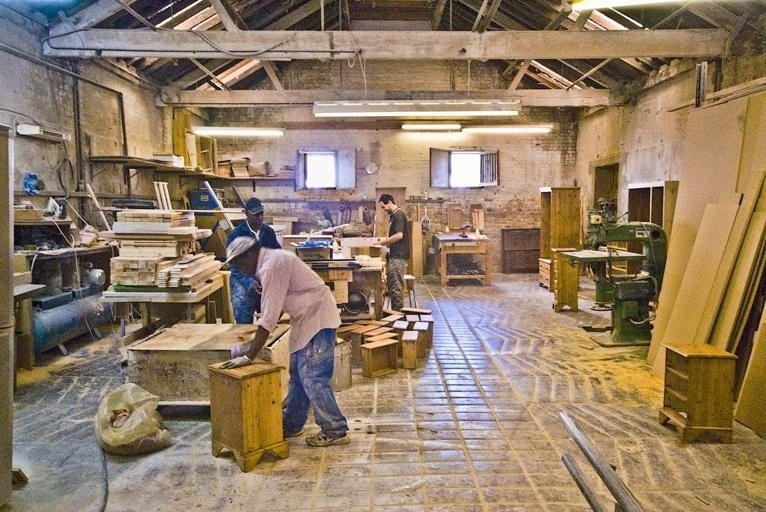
[246,198,265,215]
[222,236,256,265]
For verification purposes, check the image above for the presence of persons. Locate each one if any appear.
[219,234,350,447]
[374,193,409,310]
[225,197,282,324]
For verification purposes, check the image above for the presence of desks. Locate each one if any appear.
[285,236,387,321]
[433,232,490,287]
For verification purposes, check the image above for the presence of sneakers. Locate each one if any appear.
[306,431,350,446]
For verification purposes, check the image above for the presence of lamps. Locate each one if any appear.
[191,125,288,138]
[311,98,561,139]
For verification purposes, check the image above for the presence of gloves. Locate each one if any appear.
[218,355,252,368]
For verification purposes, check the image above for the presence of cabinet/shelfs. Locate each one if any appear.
[538,186,581,313]
[97,270,237,368]
[658,342,739,444]
[124,321,290,409]
[500,227,541,274]
